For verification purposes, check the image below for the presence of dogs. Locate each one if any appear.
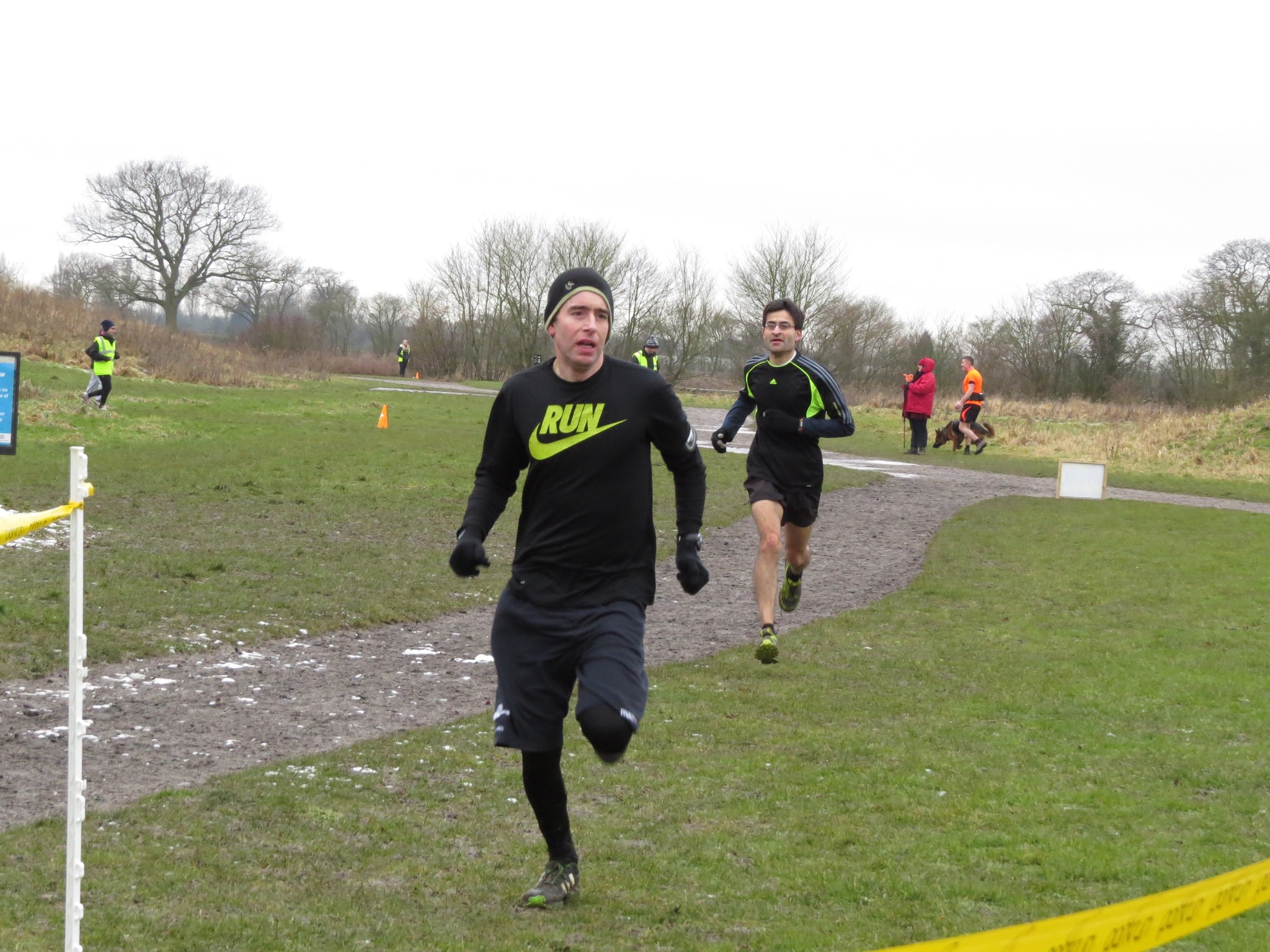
[933,420,996,451]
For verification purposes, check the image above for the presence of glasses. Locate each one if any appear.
[764,323,795,330]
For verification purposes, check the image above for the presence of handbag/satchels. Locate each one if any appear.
[964,393,984,401]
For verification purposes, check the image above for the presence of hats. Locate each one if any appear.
[918,358,925,366]
[645,334,659,348]
[544,268,613,346]
[100,320,114,332]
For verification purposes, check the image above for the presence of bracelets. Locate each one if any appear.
[677,533,703,551]
[798,418,803,434]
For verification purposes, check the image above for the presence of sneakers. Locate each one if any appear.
[754,627,779,664]
[82,392,89,401]
[917,447,926,455]
[97,406,107,410]
[904,447,917,454]
[521,860,578,909]
[974,441,987,455]
[94,399,101,406]
[400,374,405,377]
[779,559,802,611]
[963,449,970,455]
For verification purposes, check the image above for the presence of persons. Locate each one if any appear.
[449,267,710,914]
[711,298,854,666]
[81,329,103,407]
[902,357,936,456]
[82,320,120,411]
[954,355,988,455]
[396,339,411,378]
[630,335,660,374]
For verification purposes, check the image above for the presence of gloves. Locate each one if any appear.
[676,541,709,595]
[449,526,491,577]
[763,408,800,436]
[711,426,732,453]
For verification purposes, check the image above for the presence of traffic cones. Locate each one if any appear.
[377,405,388,429]
[415,372,419,379]
